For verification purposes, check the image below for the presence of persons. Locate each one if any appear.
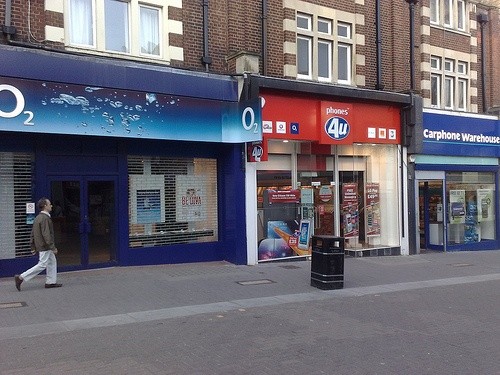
[14,198,62,291]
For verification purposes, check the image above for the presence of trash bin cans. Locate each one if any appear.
[311,235,344,289]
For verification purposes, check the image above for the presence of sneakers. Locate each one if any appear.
[15,274,23,291]
[45,283,62,288]
